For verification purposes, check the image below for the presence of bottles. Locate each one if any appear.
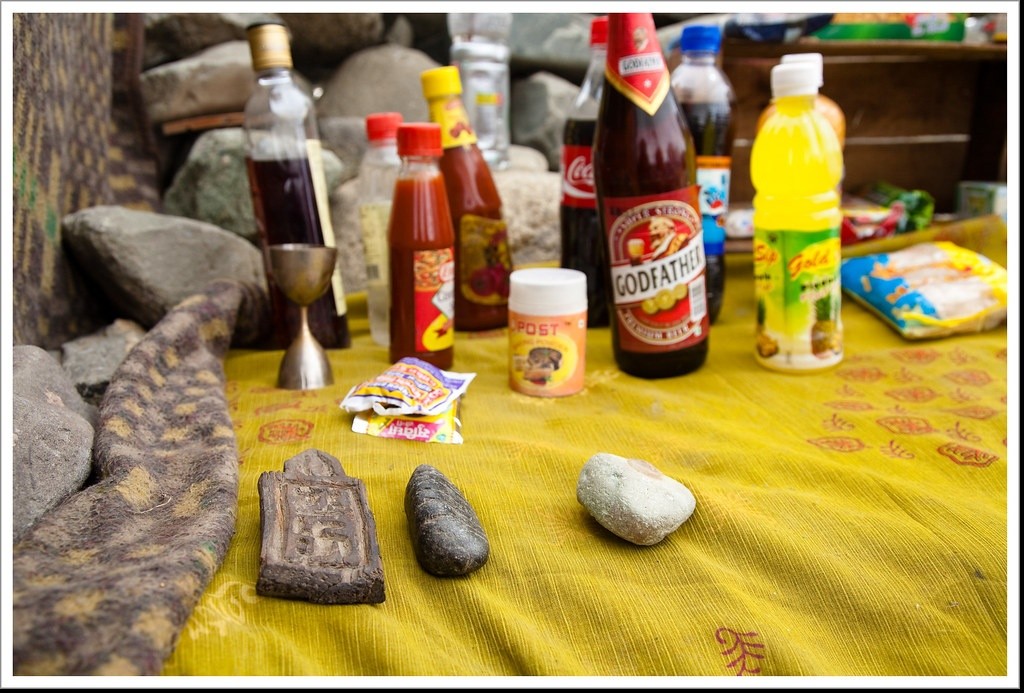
[560,13,737,380]
[749,54,846,373]
[355,65,514,372]
[244,20,348,352]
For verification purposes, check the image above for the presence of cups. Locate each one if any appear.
[507,268,589,398]
